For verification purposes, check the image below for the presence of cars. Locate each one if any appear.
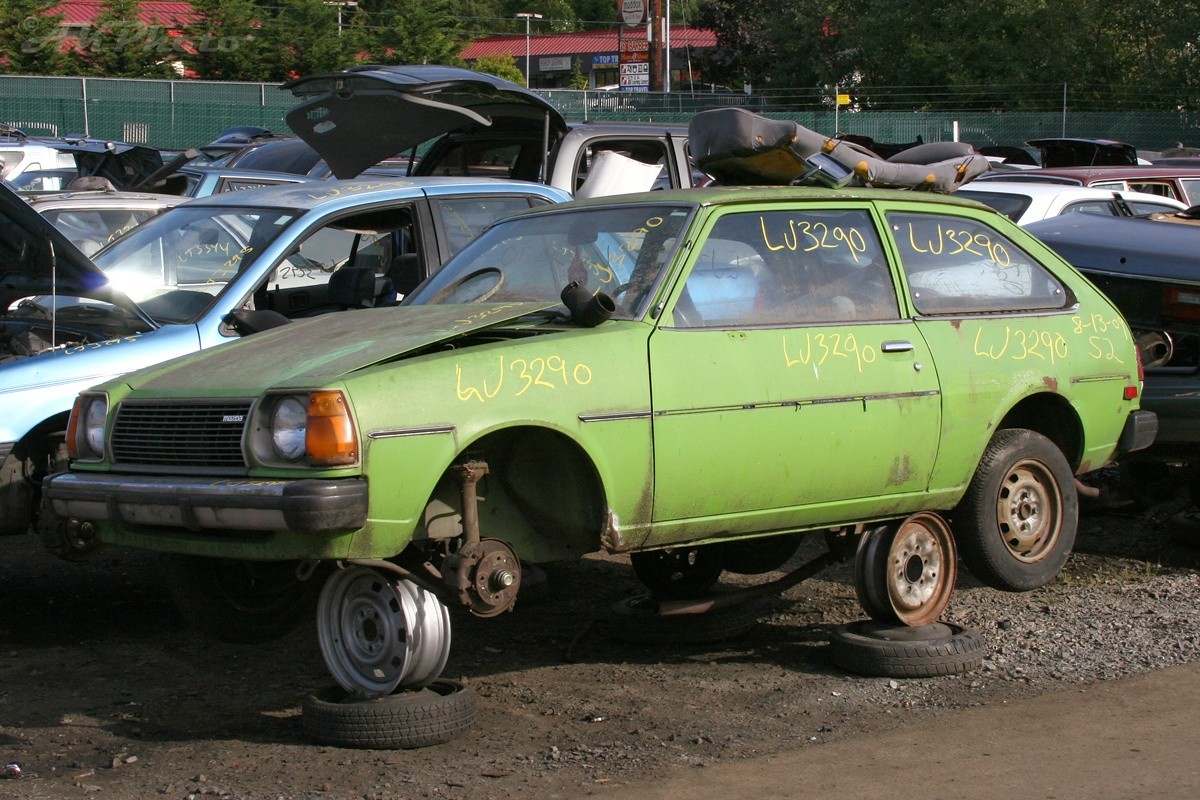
[0,67,1200,700]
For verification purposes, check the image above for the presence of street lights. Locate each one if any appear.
[516,12,543,88]
[324,0,359,47]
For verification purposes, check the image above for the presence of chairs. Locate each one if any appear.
[752,235,861,322]
